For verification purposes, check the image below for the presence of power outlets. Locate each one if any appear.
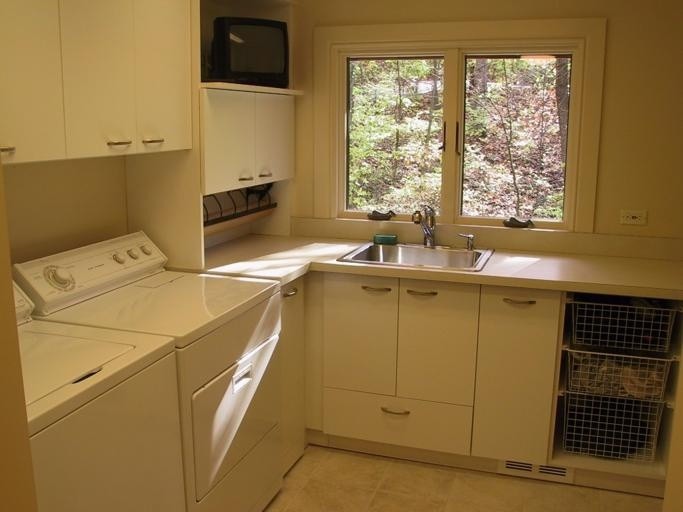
[618,206,649,228]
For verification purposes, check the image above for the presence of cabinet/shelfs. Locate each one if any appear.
[199,86,293,197]
[470,285,561,469]
[549,295,681,478]
[58,1,191,160]
[1,0,64,163]
[320,273,482,457]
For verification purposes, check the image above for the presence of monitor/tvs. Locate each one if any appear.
[209,16,290,89]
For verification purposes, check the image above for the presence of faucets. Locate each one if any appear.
[412,204,436,249]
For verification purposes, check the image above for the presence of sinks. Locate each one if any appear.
[336,241,495,272]
